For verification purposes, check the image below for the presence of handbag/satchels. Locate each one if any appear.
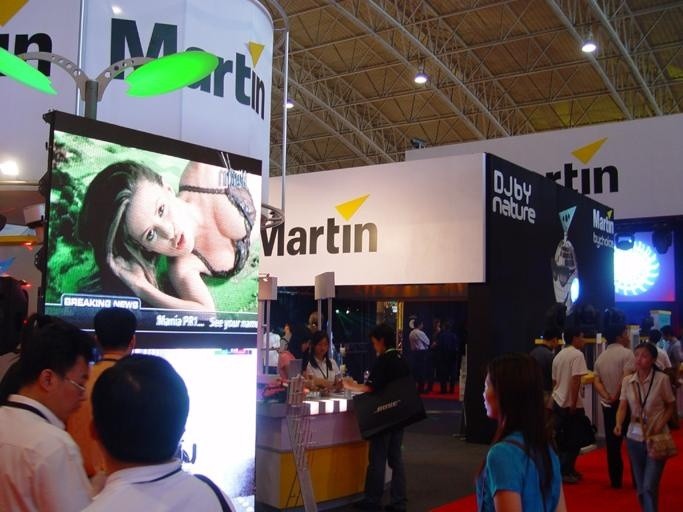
[554,406,596,449]
[645,427,678,460]
[352,374,429,442]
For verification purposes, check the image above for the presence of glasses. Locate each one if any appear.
[61,374,88,397]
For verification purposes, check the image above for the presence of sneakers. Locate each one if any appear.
[384,503,407,512]
[357,503,382,511]
[416,386,455,395]
[611,479,623,488]
[560,469,583,484]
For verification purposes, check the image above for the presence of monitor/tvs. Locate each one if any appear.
[37,109,262,351]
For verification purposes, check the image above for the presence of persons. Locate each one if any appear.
[339,324,407,512]
[475,350,567,512]
[258,312,340,386]
[78,160,256,312]
[407,317,460,395]
[0,320,94,511]
[65,307,137,477]
[80,353,235,511]
[0,313,65,399]
[532,315,683,512]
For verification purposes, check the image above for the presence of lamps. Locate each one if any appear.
[612,219,676,256]
[282,88,295,108]
[581,24,597,53]
[414,58,428,84]
